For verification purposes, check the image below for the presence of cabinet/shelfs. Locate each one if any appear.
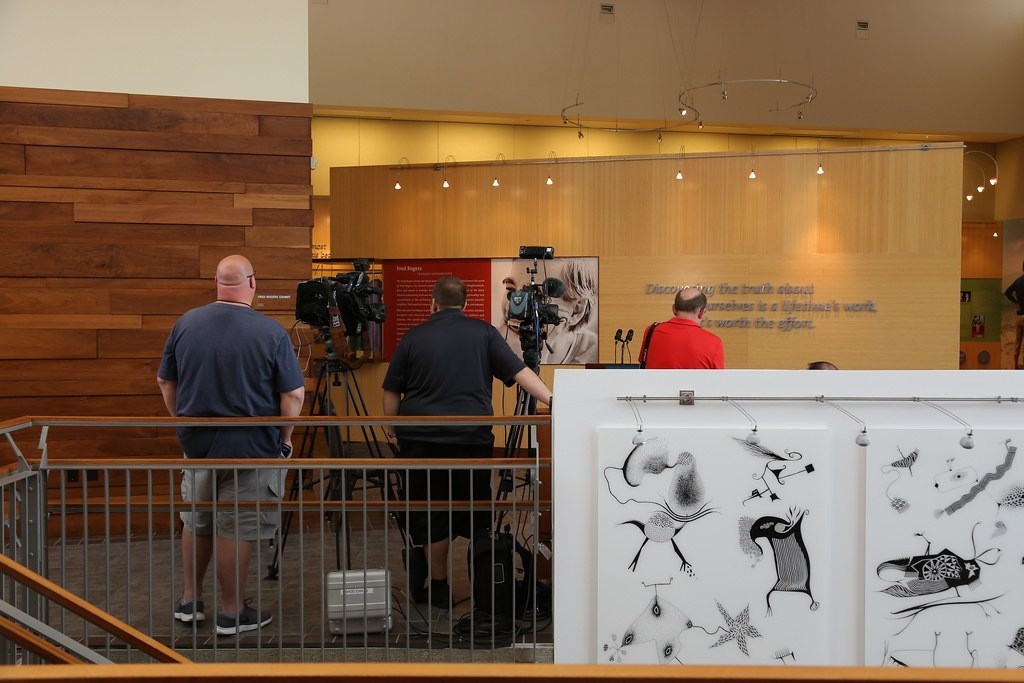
[960,220,1004,278]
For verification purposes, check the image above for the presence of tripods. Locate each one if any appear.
[263,360,412,580]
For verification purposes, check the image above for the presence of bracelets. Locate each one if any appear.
[387,433,397,438]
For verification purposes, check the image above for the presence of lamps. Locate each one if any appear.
[626,398,646,444]
[493,153,506,187]
[915,398,975,449]
[820,398,870,446]
[749,142,756,179]
[963,160,986,192]
[443,155,456,187]
[966,177,975,201]
[726,398,760,445]
[560,73,817,144]
[963,150,999,185]
[817,140,824,175]
[677,146,685,180]
[394,157,412,189]
[547,152,558,186]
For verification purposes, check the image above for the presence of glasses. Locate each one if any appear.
[246,270,257,278]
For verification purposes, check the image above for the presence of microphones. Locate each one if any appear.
[543,278,566,297]
[614,328,622,343]
[626,329,634,342]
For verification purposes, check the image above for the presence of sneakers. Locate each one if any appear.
[431,577,456,608]
[214,606,273,633]
[174,597,205,621]
[402,547,428,604]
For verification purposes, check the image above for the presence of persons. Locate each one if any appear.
[638,287,725,369]
[157,255,306,634]
[381,277,553,609]
[1005,260,1024,369]
[806,362,838,370]
[496,258,598,364]
